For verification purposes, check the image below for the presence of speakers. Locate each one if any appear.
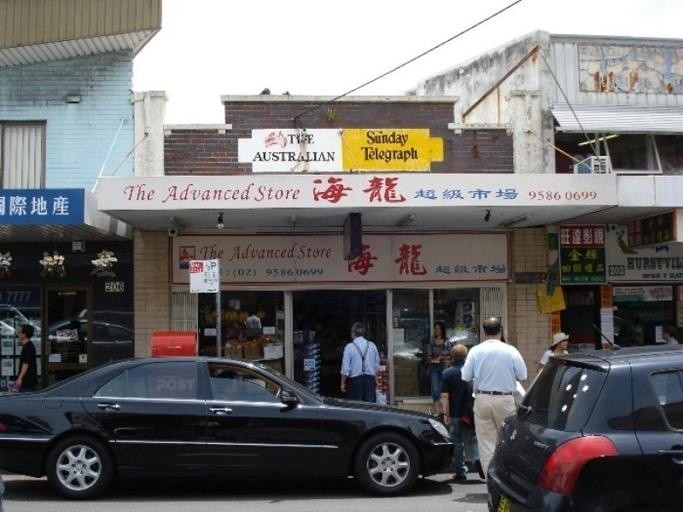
[168,227,177,237]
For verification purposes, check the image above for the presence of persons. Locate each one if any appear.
[339,322,380,403]
[15,324,37,391]
[216,370,238,401]
[439,343,485,480]
[535,332,569,374]
[423,322,452,417]
[660,324,678,345]
[460,317,527,478]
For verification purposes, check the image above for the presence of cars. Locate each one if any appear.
[392,325,481,387]
[47,319,134,365]
[0,353,456,498]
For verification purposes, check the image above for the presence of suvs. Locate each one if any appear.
[486,324,683,512]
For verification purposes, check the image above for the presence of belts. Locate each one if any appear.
[475,390,513,397]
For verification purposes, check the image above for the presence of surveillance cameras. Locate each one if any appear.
[218,215,223,224]
[485,211,491,222]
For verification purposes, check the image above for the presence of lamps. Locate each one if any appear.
[398,213,415,226]
[503,214,529,227]
[216,212,225,229]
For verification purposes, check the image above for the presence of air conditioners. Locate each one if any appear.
[574,155,610,174]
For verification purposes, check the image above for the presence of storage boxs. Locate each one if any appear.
[243,343,260,360]
[222,346,242,361]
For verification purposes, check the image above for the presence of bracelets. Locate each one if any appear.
[340,382,344,385]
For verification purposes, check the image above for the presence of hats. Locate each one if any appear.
[549,332,570,347]
